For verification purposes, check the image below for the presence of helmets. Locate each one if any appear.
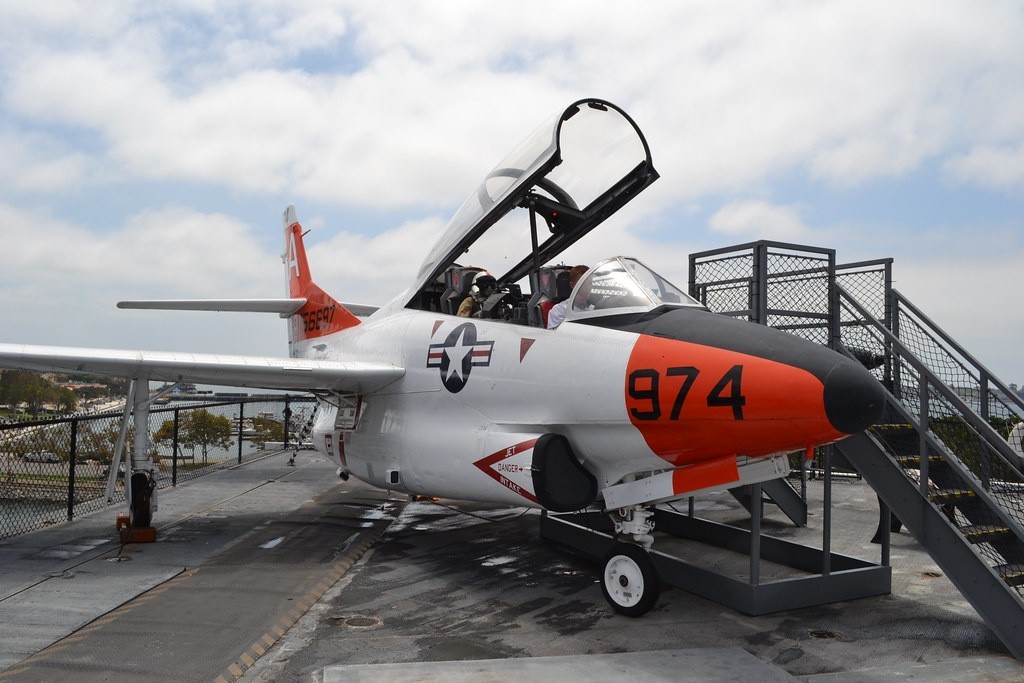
[471,270,497,292]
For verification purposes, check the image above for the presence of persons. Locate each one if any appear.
[457,270,508,319]
[546,264,598,332]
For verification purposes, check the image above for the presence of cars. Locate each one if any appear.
[23,450,60,463]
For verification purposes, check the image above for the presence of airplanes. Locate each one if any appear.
[1,95,887,621]
